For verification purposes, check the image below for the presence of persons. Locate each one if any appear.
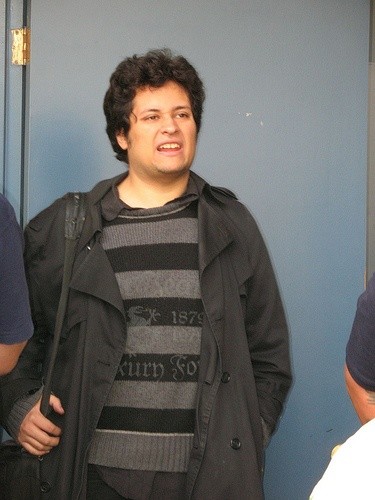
[0,45,293,500]
[0,192,34,375]
[343,269,375,427]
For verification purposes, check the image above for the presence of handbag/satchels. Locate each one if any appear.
[0,446,40,500]
